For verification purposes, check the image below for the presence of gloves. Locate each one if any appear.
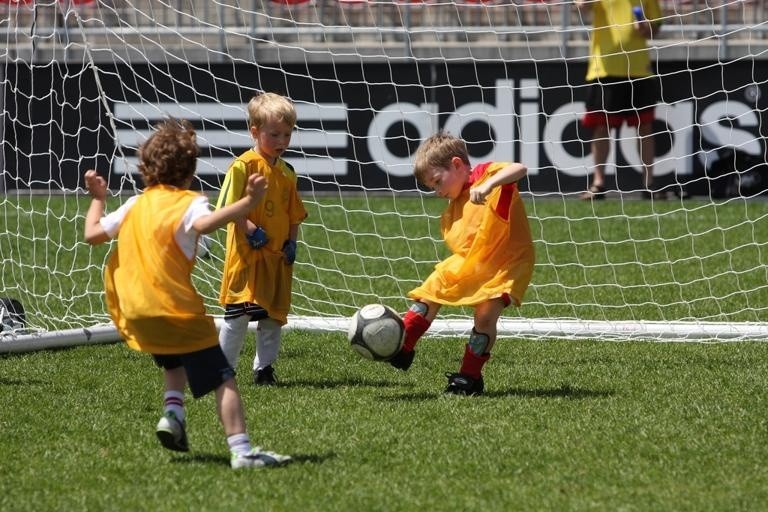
[245,226,269,249]
[281,238,297,265]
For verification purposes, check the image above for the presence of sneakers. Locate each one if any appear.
[155,410,190,452]
[444,371,484,396]
[252,365,276,386]
[390,348,415,372]
[229,446,295,469]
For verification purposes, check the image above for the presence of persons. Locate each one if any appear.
[215,89,309,388]
[572,1,669,202]
[81,112,294,471]
[386,131,535,397]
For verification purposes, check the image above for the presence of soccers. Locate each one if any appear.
[347,302,404,362]
